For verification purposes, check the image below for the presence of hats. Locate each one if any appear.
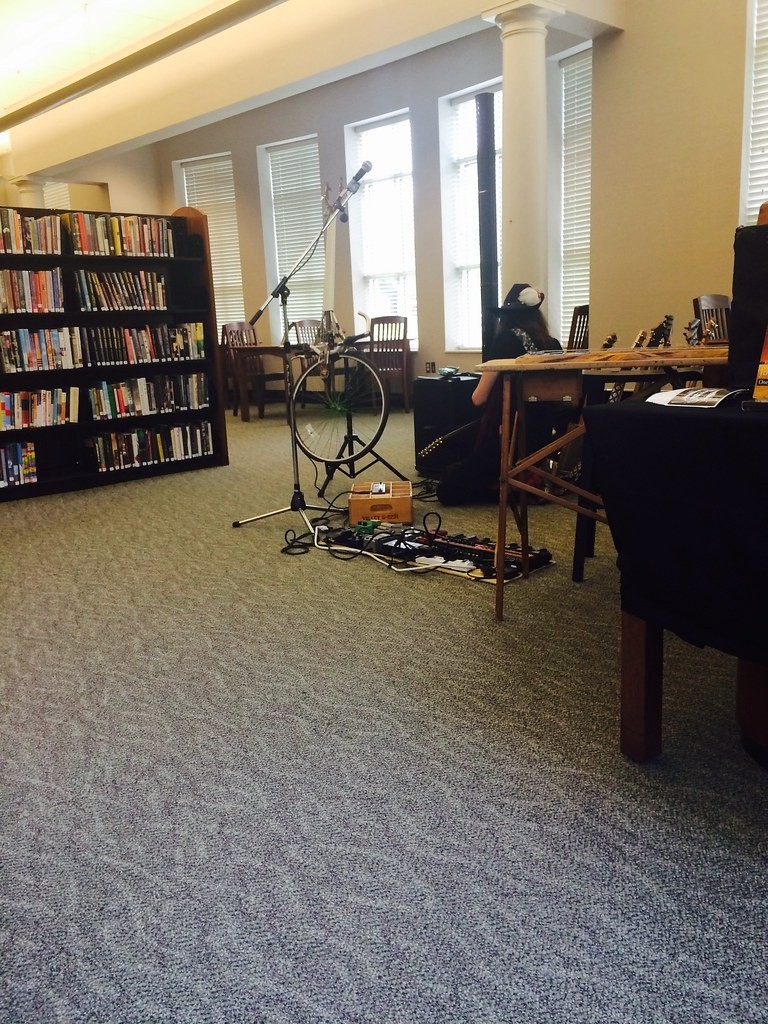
[488,283,544,318]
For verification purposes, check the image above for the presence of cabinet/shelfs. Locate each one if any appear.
[0,207,230,502]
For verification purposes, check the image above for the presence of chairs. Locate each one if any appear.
[693,294,731,344]
[369,315,410,416]
[220,322,284,419]
[567,305,589,350]
[294,320,356,408]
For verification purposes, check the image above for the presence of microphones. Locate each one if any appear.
[329,160,373,212]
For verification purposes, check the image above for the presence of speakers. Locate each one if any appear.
[413,375,483,480]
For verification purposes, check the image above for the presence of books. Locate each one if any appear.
[0,205,218,486]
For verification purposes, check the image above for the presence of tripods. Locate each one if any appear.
[317,357,411,499]
[233,182,362,540]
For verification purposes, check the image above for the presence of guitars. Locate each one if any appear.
[629,314,673,395]
[682,318,717,389]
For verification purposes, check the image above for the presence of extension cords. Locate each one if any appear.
[415,554,479,573]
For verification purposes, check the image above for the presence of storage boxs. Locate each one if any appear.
[348,481,413,527]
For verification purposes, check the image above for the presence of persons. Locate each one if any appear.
[471,284,565,507]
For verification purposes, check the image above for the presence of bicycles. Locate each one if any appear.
[286,309,391,466]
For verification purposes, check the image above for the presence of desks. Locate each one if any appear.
[229,338,414,424]
[475,356,728,621]
[580,388,768,772]
